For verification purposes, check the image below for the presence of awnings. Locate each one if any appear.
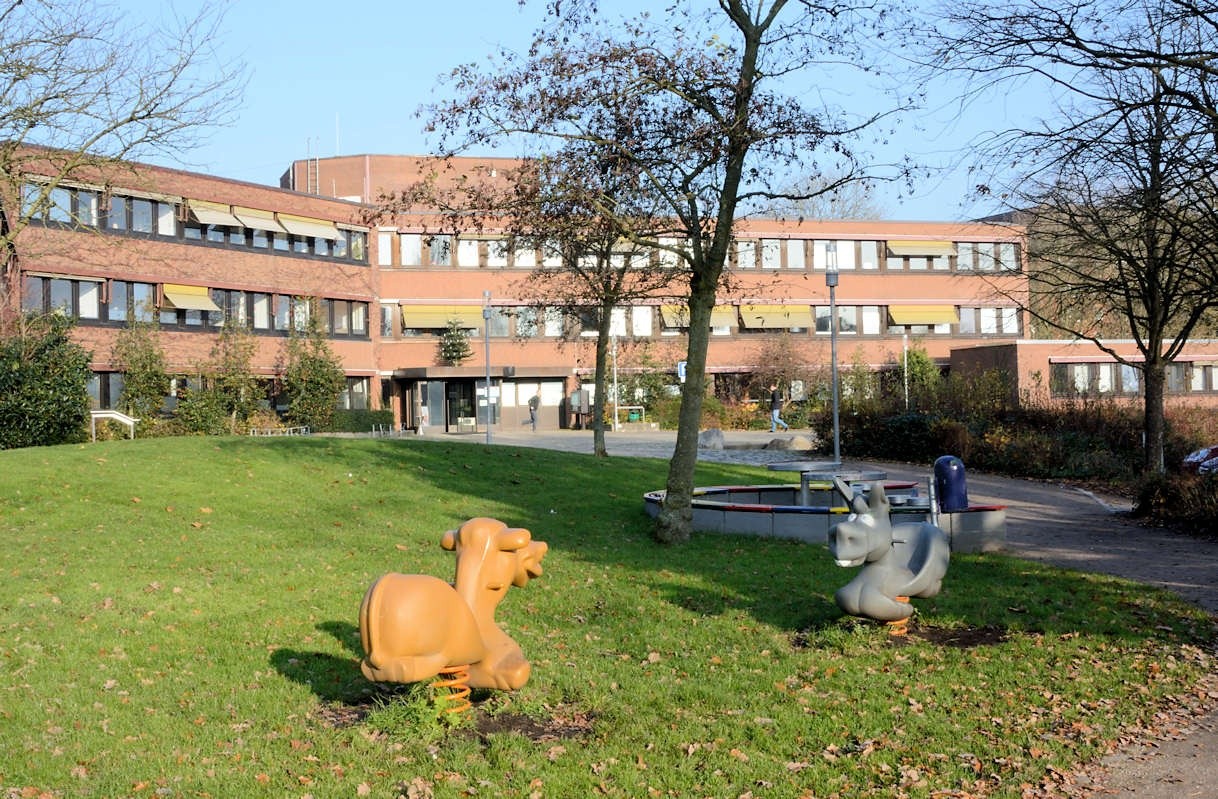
[888,305,960,324]
[163,284,221,312]
[188,199,243,226]
[888,240,958,256]
[276,213,345,240]
[400,305,483,328]
[231,205,287,233]
[660,305,740,327]
[740,305,815,328]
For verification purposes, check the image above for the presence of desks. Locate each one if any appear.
[767,460,842,507]
[802,470,888,507]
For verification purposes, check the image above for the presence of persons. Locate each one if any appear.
[768,383,788,433]
[521,390,542,433]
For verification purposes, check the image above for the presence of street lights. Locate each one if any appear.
[824,243,841,463]
[479,290,493,445]
[611,327,619,432]
[902,334,909,411]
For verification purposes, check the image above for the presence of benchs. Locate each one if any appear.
[644,479,1008,554]
